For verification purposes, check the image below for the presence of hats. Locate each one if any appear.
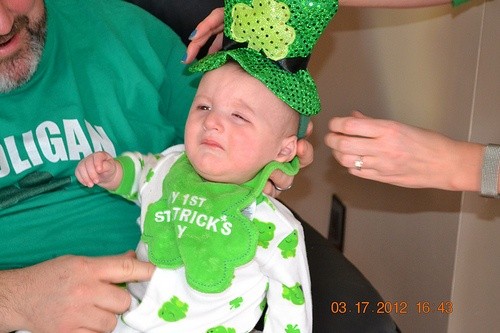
[182,0,339,119]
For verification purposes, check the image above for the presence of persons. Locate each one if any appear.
[1,1,397,332]
[74,61,314,333]
[183,0,500,199]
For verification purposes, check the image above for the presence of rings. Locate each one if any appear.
[356,155,364,171]
